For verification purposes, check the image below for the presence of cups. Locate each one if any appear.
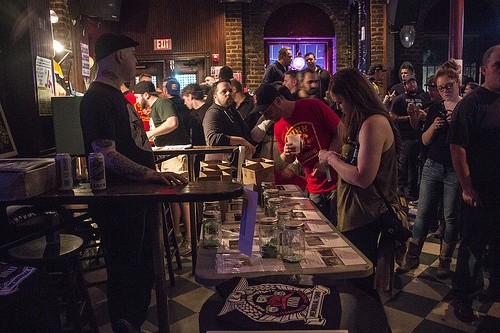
[285,134,300,153]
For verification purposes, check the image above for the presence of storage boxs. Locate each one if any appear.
[0,158,61,201]
[198,157,274,186]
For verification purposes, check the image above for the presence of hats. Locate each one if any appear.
[424,76,437,86]
[133,80,159,95]
[166,79,181,96]
[406,75,419,82]
[248,84,279,114]
[94,31,140,63]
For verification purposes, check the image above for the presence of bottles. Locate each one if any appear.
[202,200,222,248]
[258,182,306,263]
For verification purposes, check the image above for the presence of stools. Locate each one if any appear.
[161,201,182,286]
[7,234,100,333]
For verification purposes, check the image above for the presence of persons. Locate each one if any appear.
[251,84,345,228]
[318,68,400,303]
[121,48,480,238]
[447,45,500,323]
[79,32,188,333]
[402,58,463,278]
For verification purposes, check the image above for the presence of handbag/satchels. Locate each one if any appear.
[382,209,411,246]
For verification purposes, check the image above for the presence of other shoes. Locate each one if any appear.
[178,239,192,256]
[444,288,475,324]
[168,231,184,245]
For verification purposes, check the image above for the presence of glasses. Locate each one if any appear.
[437,79,457,92]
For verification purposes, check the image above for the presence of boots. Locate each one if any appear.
[437,235,459,279]
[396,237,427,273]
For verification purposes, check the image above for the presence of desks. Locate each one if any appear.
[0,179,243,281]
[191,184,376,333]
[65,144,237,275]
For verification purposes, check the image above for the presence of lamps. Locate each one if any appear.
[50,9,58,23]
